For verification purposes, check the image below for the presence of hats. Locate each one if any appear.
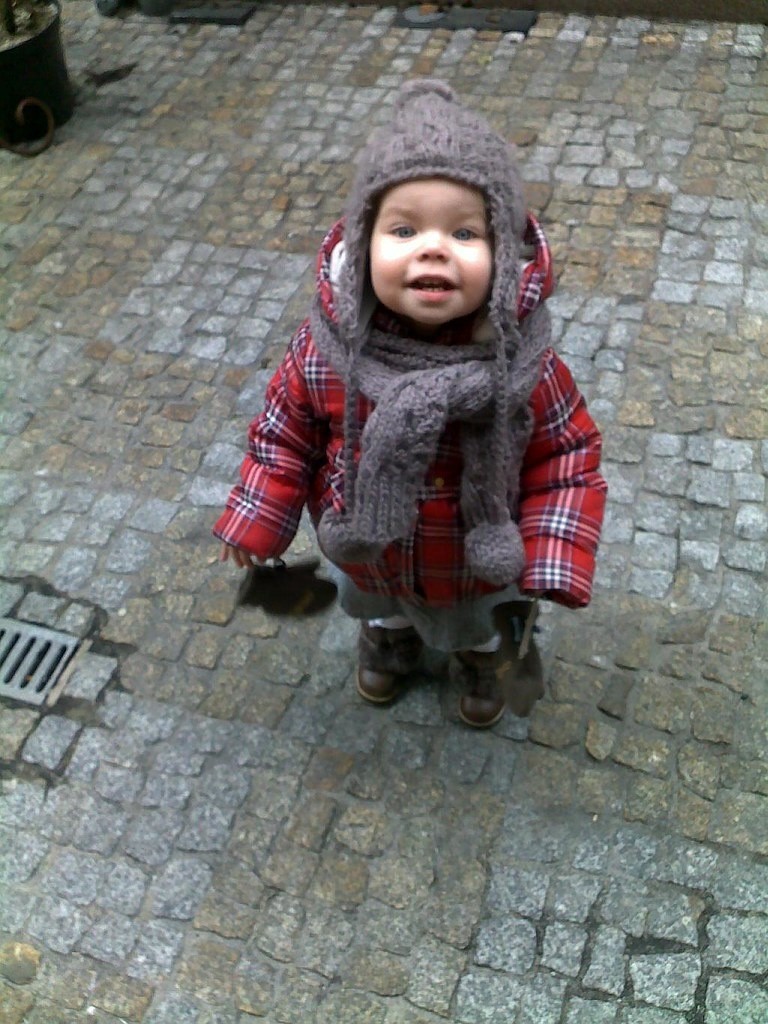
[317,78,527,584]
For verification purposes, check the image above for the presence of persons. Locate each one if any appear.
[212,79,609,728]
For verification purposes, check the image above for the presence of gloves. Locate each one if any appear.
[480,602,547,718]
[236,559,338,616]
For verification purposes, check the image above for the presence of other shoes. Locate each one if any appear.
[354,621,422,702]
[449,650,507,727]
